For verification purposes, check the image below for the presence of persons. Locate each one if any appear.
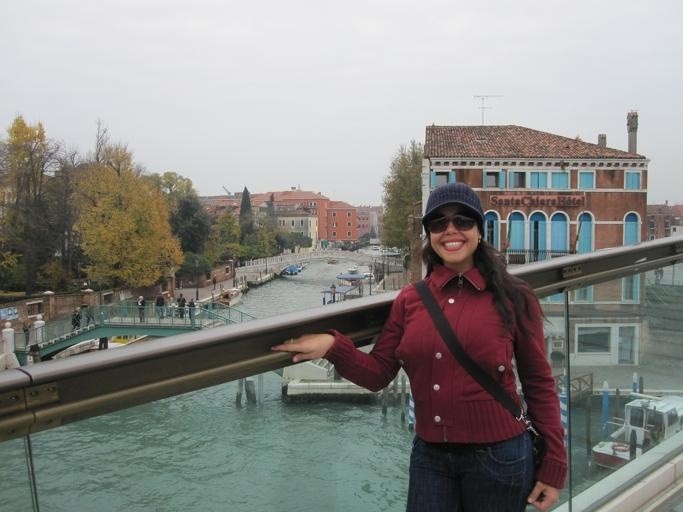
[177,293,186,318]
[23,321,31,346]
[137,296,146,323]
[86,302,94,325]
[155,292,165,319]
[271,182,568,512]
[189,298,194,319]
[72,306,82,330]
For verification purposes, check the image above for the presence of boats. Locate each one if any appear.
[348,265,358,273]
[328,260,337,264]
[372,244,379,250]
[592,394,683,471]
[217,289,243,308]
[286,262,304,275]
[52,334,147,360]
[364,272,376,284]
[320,274,365,305]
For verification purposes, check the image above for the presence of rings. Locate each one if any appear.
[291,337,293,344]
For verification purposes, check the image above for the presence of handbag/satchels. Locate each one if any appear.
[524,419,547,473]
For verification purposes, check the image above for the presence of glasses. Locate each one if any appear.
[426,210,476,234]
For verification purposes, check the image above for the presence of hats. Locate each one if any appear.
[421,183,485,226]
